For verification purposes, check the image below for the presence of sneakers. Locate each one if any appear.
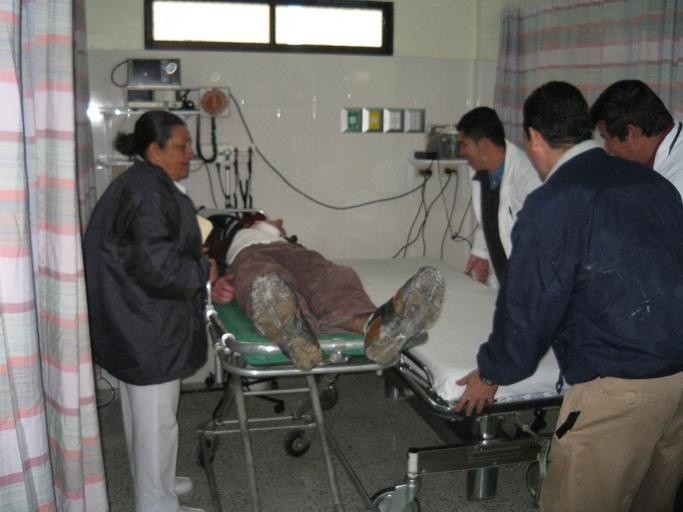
[174,475,193,496]
[178,505,206,512]
[249,271,325,373]
[363,266,446,365]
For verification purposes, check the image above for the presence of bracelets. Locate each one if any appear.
[478,374,492,386]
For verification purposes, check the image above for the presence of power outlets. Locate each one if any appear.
[413,164,458,178]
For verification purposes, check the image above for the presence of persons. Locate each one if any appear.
[591,79,683,199]
[205,212,446,372]
[453,81,683,512]
[456,109,544,291]
[82,110,217,512]
